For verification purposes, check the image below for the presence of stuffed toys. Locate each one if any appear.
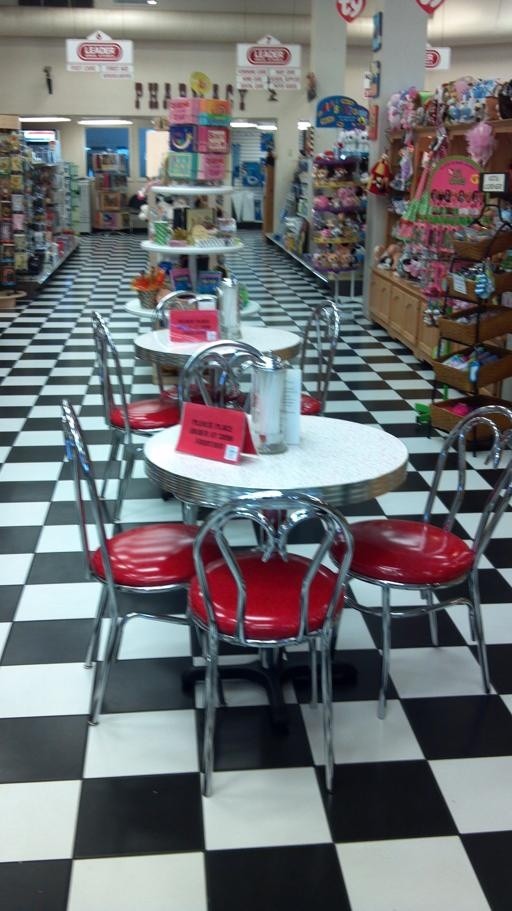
[309,128,371,274]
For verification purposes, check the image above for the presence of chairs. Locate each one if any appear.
[58,398,227,727]
[186,488,357,794]
[330,404,512,724]
[301,299,344,410]
[148,290,211,389]
[89,311,183,515]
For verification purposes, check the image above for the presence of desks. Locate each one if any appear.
[143,413,412,544]
[128,323,301,389]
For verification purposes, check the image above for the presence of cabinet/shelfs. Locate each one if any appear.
[124,125,263,324]
[367,125,512,396]
[267,128,365,296]
[1,126,78,309]
